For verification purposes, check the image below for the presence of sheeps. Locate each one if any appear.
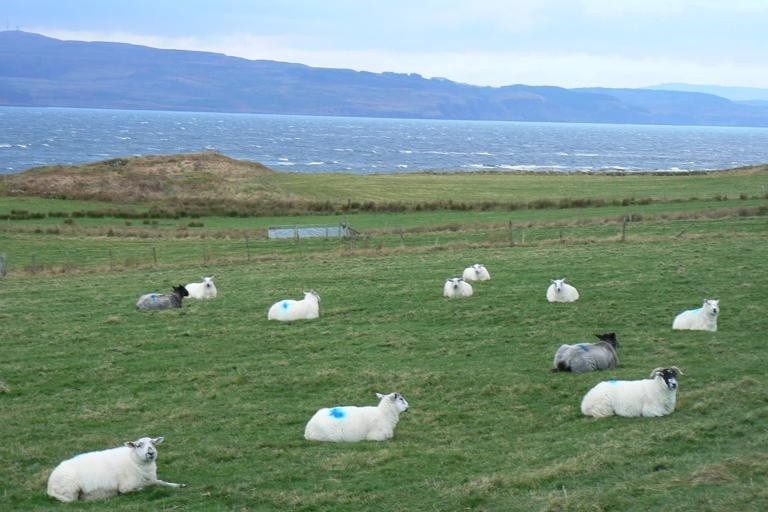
[268,289,321,321]
[549,332,625,372]
[47,437,187,502]
[304,391,409,442]
[546,277,580,303]
[580,366,687,417]
[183,276,217,300]
[443,278,473,298]
[136,284,189,310]
[672,298,720,332]
[462,263,491,281]
